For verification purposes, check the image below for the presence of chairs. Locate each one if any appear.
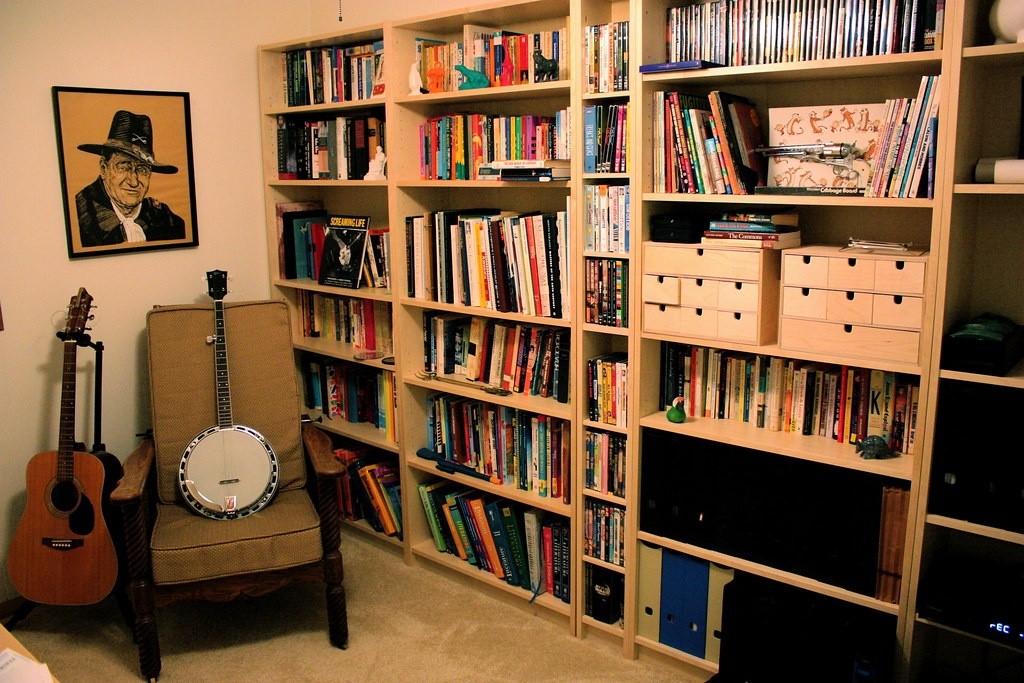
[111,298,348,683]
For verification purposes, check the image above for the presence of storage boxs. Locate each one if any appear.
[777,241,927,365]
[641,240,779,347]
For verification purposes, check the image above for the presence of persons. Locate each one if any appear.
[586,292,591,308]
[371,146,383,172]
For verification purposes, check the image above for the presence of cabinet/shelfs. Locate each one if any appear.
[257,0,1024,683]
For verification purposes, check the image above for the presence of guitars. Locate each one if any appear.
[5,286,119,606]
[177,268,280,521]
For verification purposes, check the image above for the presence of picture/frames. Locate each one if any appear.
[52,85,200,260]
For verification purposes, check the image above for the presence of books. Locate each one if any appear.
[640,0,944,456]
[277,19,628,604]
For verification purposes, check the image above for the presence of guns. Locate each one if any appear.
[754,141,872,188]
[417,446,502,484]
